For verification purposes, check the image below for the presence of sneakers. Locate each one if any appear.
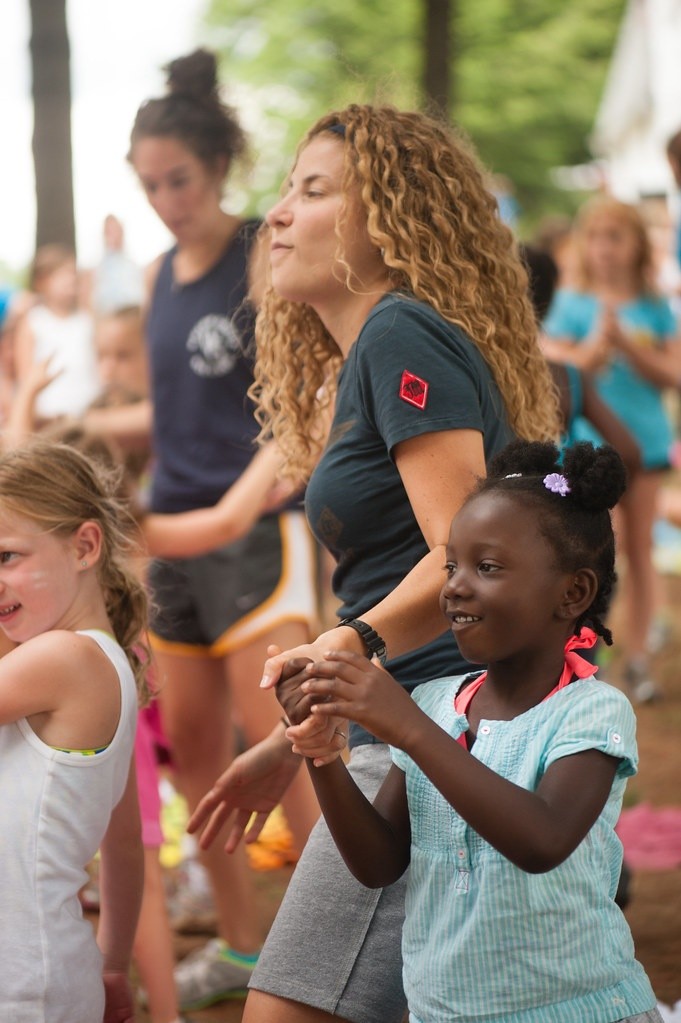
[137,937,261,1010]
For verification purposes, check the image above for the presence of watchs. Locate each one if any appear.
[337,617,387,666]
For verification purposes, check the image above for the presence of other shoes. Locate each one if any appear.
[621,657,656,703]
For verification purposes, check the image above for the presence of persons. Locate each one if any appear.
[516,133,681,701]
[0,211,152,478]
[94,48,322,1012]
[185,118,560,1023]
[0,440,180,1023]
[276,440,665,1023]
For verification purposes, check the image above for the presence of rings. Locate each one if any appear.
[335,731,347,739]
